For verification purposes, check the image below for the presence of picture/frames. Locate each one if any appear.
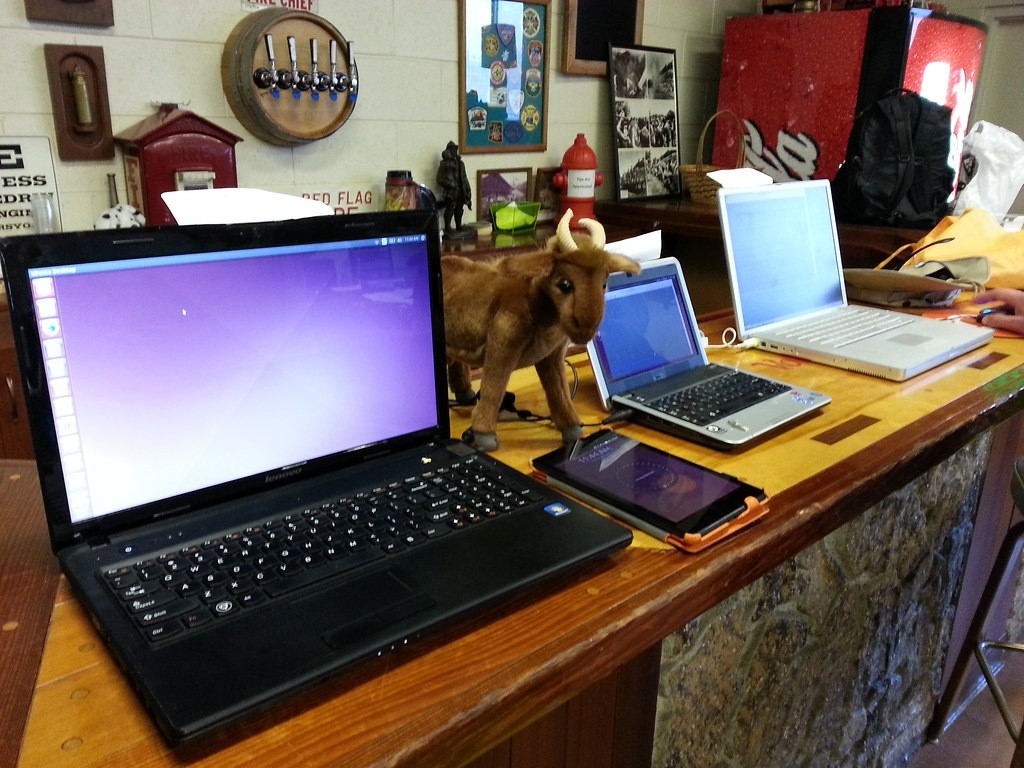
[458,0,553,156]
[534,167,562,208]
[603,40,682,203]
[477,167,534,221]
[563,0,645,76]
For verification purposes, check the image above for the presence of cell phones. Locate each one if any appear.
[531,428,767,540]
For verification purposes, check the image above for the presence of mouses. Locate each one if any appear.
[977,305,1015,323]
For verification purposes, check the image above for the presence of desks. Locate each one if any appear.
[0,306,1024,768]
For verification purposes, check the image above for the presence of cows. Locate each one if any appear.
[439,207,640,451]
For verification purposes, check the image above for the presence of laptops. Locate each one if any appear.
[717,178,996,382]
[0,208,634,759]
[585,257,832,445]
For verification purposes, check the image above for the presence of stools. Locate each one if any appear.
[927,460,1024,768]
